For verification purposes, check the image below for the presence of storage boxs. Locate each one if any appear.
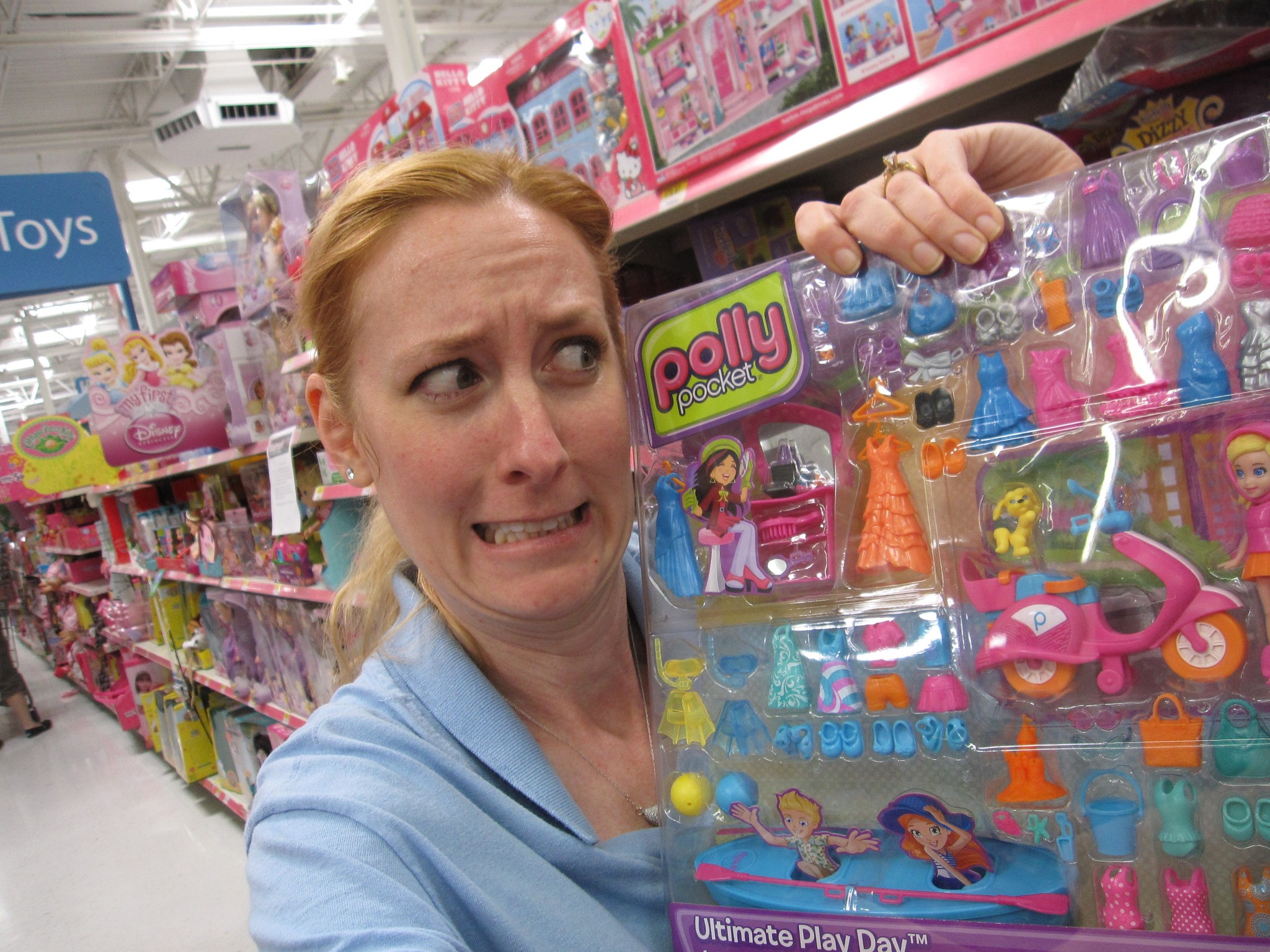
[6,1,1269,807]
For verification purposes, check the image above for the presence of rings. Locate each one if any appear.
[881,151,925,196]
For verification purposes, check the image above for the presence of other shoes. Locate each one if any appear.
[26,720,51,738]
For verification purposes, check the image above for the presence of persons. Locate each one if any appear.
[1217,420,1270,684]
[39,559,83,678]
[0,625,52,749]
[247,192,286,292]
[212,601,313,708]
[241,119,1085,952]
[296,462,334,566]
[163,508,201,560]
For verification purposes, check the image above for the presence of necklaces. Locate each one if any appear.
[495,611,663,826]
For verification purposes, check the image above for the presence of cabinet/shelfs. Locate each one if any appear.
[10,485,110,695]
[98,428,370,826]
[279,1,1179,499]
